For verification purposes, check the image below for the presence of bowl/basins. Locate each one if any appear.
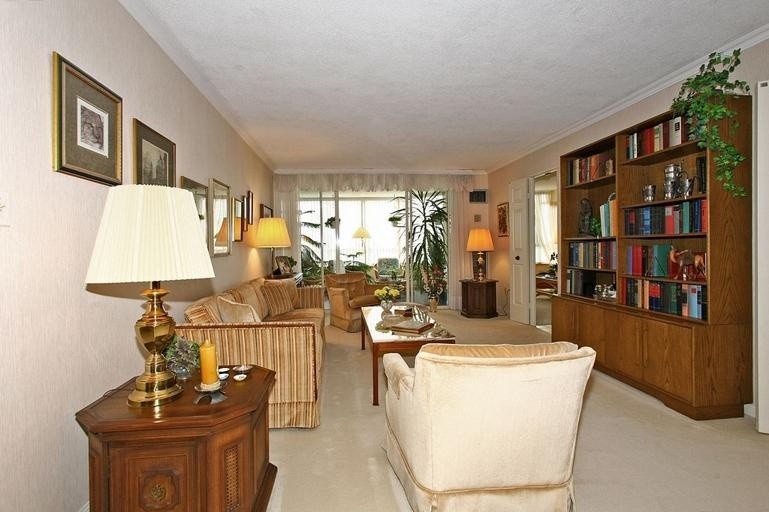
[233,364,254,375]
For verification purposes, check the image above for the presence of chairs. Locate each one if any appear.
[324,272,389,333]
[382,341,597,512]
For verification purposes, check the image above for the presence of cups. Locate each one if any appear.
[642,184,656,204]
[681,178,695,197]
[663,182,676,199]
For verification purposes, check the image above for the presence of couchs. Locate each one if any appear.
[173,277,325,430]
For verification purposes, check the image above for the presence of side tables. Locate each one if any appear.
[460,278,500,319]
[271,272,303,287]
[76,365,277,512]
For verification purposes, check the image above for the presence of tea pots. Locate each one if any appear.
[663,163,688,198]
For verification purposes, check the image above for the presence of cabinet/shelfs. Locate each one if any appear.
[551,94,753,422]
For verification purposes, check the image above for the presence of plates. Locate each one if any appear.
[233,374,248,381]
[218,373,228,381]
[219,366,230,373]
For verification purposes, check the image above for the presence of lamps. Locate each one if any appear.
[352,227,373,252]
[464,228,495,281]
[256,217,291,278]
[85,185,216,408]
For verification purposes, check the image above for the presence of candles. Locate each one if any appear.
[199,340,218,384]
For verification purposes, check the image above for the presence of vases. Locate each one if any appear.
[428,294,437,312]
[170,363,192,380]
[381,310,392,320]
[381,301,392,312]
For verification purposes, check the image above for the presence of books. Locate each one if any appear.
[390,320,434,334]
[564,109,709,320]
[392,328,435,338]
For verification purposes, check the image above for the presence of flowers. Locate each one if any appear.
[162,336,200,373]
[375,321,390,333]
[420,260,447,304]
[375,286,400,303]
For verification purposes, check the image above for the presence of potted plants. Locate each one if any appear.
[325,217,340,229]
[288,257,297,268]
[671,45,750,199]
[388,216,401,226]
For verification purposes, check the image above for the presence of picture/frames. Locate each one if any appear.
[231,196,243,242]
[497,202,510,237]
[260,203,273,218]
[52,49,125,187]
[243,195,249,232]
[248,190,254,225]
[180,174,209,221]
[131,115,177,187]
[209,177,231,257]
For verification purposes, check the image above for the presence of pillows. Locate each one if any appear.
[184,277,302,323]
[418,342,578,358]
